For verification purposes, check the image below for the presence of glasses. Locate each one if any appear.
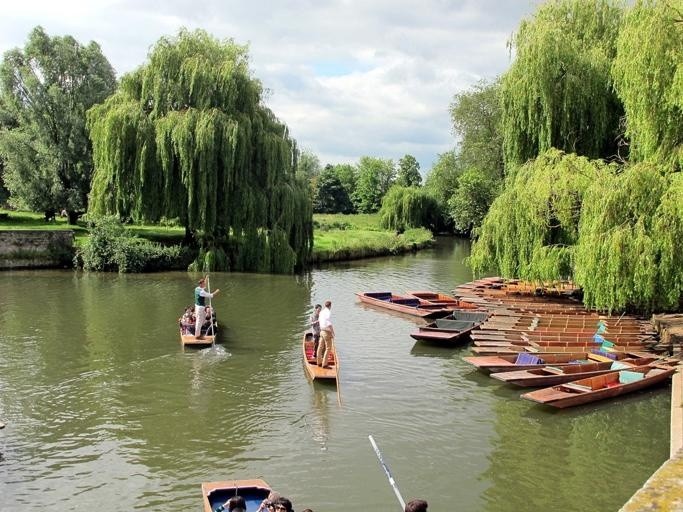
[265,502,286,510]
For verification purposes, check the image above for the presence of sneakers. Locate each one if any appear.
[318,363,332,369]
[312,352,316,359]
[195,336,206,340]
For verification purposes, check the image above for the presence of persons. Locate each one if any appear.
[214,493,295,512]
[310,301,335,369]
[179,279,220,340]
[405,500,428,512]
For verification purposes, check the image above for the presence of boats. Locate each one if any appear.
[199,476,280,512]
[178,308,217,345]
[300,358,337,394]
[302,328,341,383]
[180,339,208,354]
